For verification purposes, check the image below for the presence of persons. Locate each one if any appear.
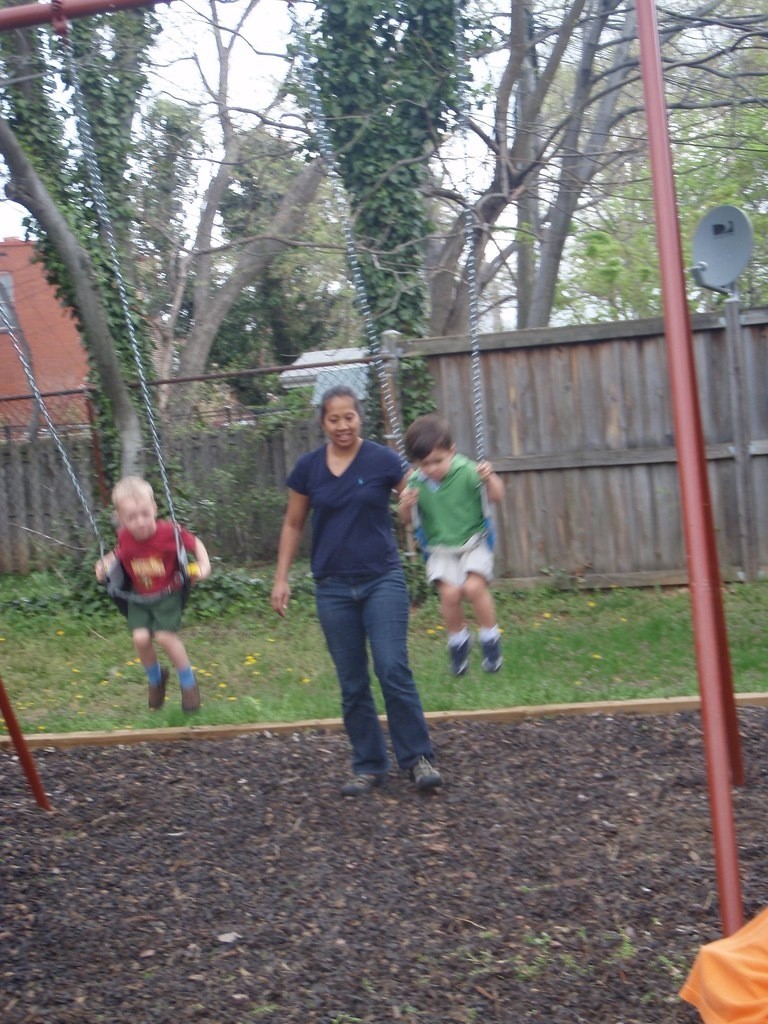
[271,386,441,798]
[397,415,503,677]
[94,475,212,712]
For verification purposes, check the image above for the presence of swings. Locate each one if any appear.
[0,0,192,619]
[286,0,494,563]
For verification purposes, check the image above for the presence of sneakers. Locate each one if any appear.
[449,636,472,676]
[481,633,502,671]
[341,771,380,795]
[410,756,441,787]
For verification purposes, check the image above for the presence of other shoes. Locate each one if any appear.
[148,665,170,710]
[180,675,201,711]
